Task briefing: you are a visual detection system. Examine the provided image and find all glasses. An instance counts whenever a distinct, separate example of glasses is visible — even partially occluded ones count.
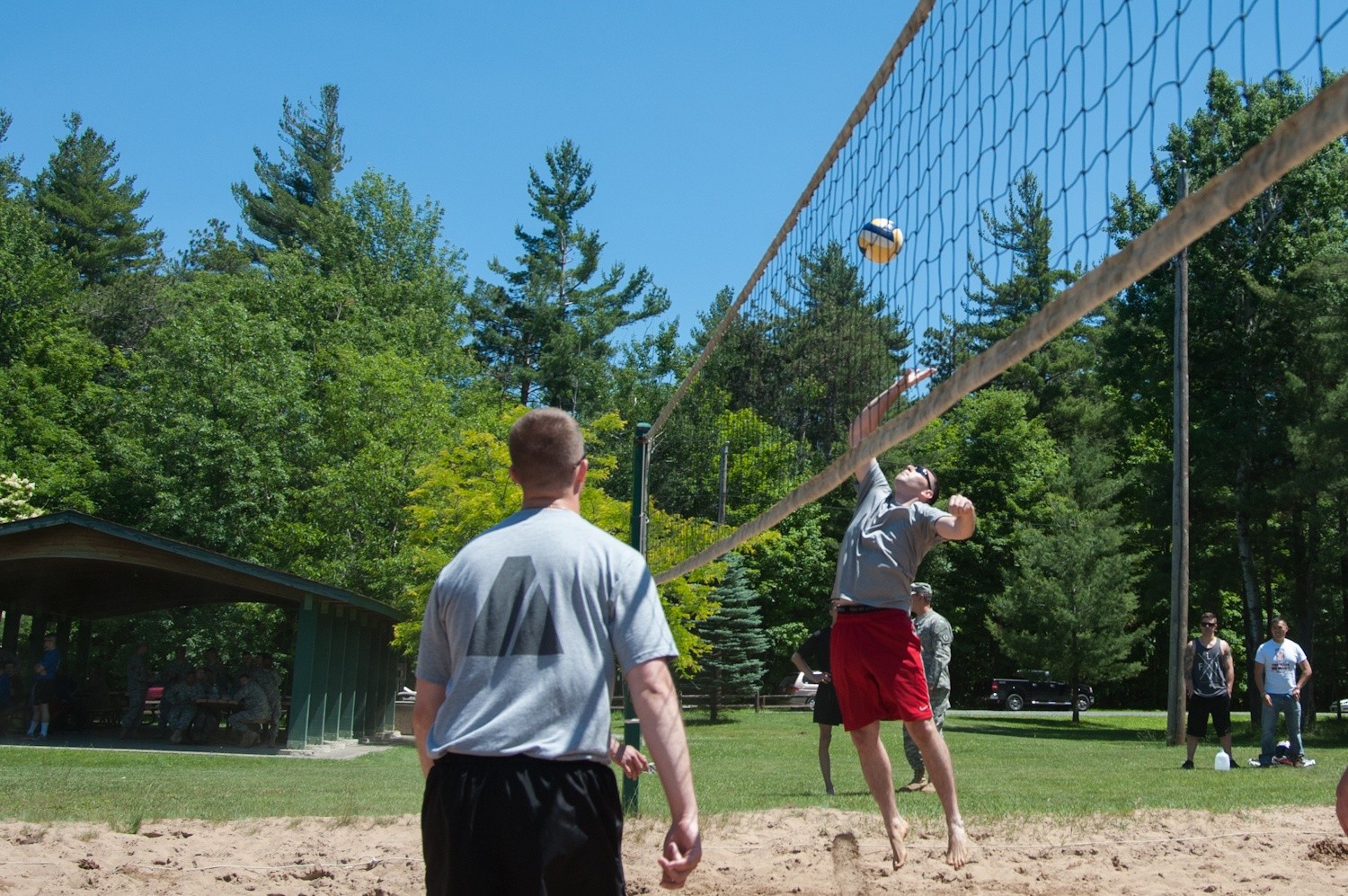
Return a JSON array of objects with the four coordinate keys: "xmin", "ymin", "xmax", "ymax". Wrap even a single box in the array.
[
  {"xmin": 913, "ymin": 464, "xmax": 931, "ymax": 490},
  {"xmin": 1201, "ymin": 622, "xmax": 1216, "ymax": 627}
]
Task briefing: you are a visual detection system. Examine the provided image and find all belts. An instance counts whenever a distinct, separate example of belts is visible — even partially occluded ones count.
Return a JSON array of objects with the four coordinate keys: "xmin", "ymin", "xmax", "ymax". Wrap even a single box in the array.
[{"xmin": 837, "ymin": 604, "xmax": 883, "ymax": 614}]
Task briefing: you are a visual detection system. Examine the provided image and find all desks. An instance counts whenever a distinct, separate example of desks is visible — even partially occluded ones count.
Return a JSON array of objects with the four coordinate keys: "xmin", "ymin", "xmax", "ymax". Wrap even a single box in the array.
[{"xmin": 195, "ymin": 699, "xmax": 237, "ymax": 742}]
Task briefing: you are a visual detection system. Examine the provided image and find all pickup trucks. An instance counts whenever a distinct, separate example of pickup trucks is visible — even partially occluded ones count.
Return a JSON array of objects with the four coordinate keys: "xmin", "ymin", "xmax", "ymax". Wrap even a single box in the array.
[{"xmin": 973, "ymin": 668, "xmax": 1096, "ymax": 711}]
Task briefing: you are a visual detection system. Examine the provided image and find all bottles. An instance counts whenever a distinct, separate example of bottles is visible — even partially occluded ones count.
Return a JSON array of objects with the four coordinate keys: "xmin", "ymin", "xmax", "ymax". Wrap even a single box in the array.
[
  {"xmin": 1214, "ymin": 748, "xmax": 1231, "ymax": 771},
  {"xmin": 209, "ymin": 681, "xmax": 233, "ymax": 699}
]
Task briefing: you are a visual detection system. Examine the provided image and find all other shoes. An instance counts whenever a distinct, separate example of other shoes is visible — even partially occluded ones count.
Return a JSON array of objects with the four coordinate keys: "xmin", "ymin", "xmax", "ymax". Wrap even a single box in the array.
[
  {"xmin": 1230, "ymin": 760, "xmax": 1240, "ymax": 768},
  {"xmin": 1182, "ymin": 760, "xmax": 1194, "ymax": 770},
  {"xmin": 1294, "ymin": 756, "xmax": 1306, "ymax": 767},
  {"xmin": 1259, "ymin": 764, "xmax": 1270, "ymax": 768}
]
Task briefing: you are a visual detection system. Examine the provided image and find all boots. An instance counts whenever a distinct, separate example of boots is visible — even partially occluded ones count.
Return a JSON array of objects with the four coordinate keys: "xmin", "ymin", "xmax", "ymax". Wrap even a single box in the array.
[
  {"xmin": 899, "ymin": 769, "xmax": 928, "ymax": 792},
  {"xmin": 921, "ymin": 781, "xmax": 937, "ymax": 792}
]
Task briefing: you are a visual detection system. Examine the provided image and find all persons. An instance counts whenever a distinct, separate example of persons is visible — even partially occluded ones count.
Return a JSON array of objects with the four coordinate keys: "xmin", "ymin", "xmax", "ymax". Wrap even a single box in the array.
[
  {"xmin": 0, "ymin": 631, "xmax": 282, "ymax": 750},
  {"xmin": 827, "ymin": 364, "xmax": 977, "ymax": 871},
  {"xmin": 1335, "ymin": 766, "xmax": 1348, "ymax": 836},
  {"xmin": 792, "ymin": 581, "xmax": 955, "ymax": 795},
  {"xmin": 1181, "ymin": 611, "xmax": 1243, "ymax": 770},
  {"xmin": 1257, "ymin": 614, "xmax": 1316, "ymax": 767},
  {"xmin": 414, "ymin": 407, "xmax": 702, "ymax": 896}
]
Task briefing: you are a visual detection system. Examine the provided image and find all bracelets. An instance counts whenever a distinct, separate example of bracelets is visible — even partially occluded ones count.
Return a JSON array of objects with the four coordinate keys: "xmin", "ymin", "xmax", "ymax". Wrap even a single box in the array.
[{"xmin": 1261, "ymin": 691, "xmax": 1267, "ymax": 698}]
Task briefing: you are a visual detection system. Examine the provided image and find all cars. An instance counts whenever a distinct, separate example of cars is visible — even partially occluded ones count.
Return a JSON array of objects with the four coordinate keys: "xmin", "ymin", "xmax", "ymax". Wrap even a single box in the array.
[
  {"xmin": 775, "ymin": 670, "xmax": 823, "ymax": 711},
  {"xmin": 396, "ymin": 686, "xmax": 417, "ymax": 702},
  {"xmin": 1329, "ymin": 697, "xmax": 1348, "ymax": 713}
]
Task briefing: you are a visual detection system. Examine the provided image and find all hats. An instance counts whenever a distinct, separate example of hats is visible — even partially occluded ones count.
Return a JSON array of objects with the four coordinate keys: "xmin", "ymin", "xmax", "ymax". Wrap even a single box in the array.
[{"xmin": 910, "ymin": 583, "xmax": 932, "ymax": 599}]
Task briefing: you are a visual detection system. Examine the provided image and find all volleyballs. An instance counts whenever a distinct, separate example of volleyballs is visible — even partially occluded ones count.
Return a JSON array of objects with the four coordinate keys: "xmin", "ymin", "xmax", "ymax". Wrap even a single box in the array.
[{"xmin": 860, "ymin": 220, "xmax": 901, "ymax": 261}]
